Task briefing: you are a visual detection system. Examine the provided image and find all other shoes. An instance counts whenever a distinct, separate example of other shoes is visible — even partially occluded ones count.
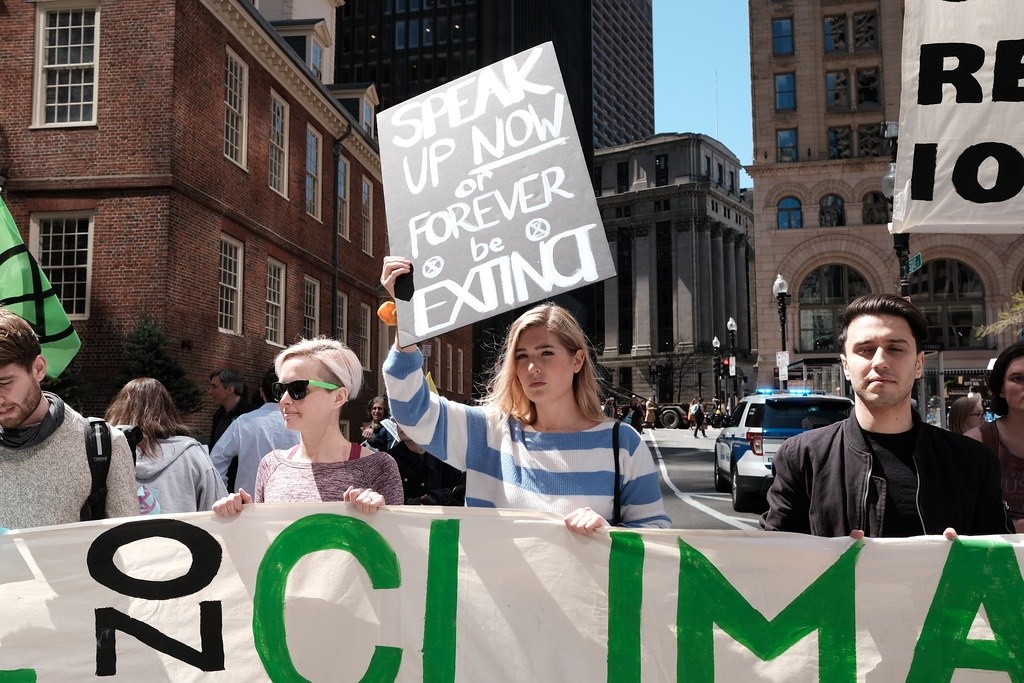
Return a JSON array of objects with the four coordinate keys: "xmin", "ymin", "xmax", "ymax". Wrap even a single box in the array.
[
  {"xmin": 651, "ymin": 426, "xmax": 655, "ymax": 430},
  {"xmin": 693, "ymin": 435, "xmax": 699, "ymax": 439},
  {"xmin": 702, "ymin": 435, "xmax": 708, "ymax": 439}
]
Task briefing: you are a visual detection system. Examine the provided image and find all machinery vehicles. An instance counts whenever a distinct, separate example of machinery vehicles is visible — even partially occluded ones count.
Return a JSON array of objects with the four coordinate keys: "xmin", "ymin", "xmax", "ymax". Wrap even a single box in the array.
[{"xmin": 638, "ymin": 399, "xmax": 689, "ymax": 428}]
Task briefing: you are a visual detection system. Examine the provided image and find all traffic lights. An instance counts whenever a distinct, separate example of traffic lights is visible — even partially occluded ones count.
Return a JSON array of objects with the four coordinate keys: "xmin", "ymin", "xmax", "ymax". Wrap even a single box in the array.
[{"xmin": 722, "ymin": 360, "xmax": 729, "ymax": 377}]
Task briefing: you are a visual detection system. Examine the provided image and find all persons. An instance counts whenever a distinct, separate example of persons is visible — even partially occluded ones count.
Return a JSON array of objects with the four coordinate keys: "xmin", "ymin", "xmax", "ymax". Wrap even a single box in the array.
[
  {"xmin": 0, "ymin": 307, "xmax": 140, "ymax": 530},
  {"xmin": 380, "ymin": 256, "xmax": 673, "ymax": 536},
  {"xmin": 209, "ymin": 368, "xmax": 300, "ymax": 503},
  {"xmin": 212, "ymin": 339, "xmax": 404, "ymax": 516},
  {"xmin": 949, "ymin": 341, "xmax": 1024, "ymax": 533},
  {"xmin": 104, "ymin": 377, "xmax": 229, "ymax": 515},
  {"xmin": 759, "ymin": 292, "xmax": 1016, "ymax": 539},
  {"xmin": 688, "ymin": 397, "xmax": 708, "ymax": 438},
  {"xmin": 360, "ymin": 398, "xmax": 467, "ymax": 506},
  {"xmin": 604, "ymin": 395, "xmax": 656, "ymax": 435}
]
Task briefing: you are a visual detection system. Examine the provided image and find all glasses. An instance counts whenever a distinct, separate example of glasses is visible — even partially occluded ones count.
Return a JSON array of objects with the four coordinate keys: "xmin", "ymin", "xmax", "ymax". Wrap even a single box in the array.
[
  {"xmin": 272, "ymin": 379, "xmax": 348, "ymax": 403},
  {"xmin": 969, "ymin": 412, "xmax": 987, "ymax": 419}
]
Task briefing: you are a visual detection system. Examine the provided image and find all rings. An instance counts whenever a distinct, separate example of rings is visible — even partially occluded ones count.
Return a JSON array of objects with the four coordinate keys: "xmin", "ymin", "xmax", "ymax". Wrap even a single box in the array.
[{"xmin": 367, "ymin": 496, "xmax": 374, "ymax": 502}]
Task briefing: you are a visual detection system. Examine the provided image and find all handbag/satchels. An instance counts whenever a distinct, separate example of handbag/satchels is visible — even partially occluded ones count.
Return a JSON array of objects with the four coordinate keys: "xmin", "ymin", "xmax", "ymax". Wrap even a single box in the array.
[
  {"xmin": 691, "ymin": 404, "xmax": 700, "ymax": 416},
  {"xmin": 688, "ymin": 412, "xmax": 696, "ymax": 421}
]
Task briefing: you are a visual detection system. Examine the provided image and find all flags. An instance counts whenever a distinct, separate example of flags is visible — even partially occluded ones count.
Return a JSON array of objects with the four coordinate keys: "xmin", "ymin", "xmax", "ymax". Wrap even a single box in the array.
[{"xmin": 0, "ymin": 196, "xmax": 82, "ymax": 380}]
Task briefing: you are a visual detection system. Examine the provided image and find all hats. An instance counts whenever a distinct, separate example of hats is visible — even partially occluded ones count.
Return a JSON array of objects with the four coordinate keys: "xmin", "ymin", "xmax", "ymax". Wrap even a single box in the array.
[{"xmin": 609, "ymin": 397, "xmax": 614, "ymax": 401}]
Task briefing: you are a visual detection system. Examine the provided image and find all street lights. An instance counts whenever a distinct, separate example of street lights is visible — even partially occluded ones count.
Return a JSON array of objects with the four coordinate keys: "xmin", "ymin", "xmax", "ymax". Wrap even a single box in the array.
[
  {"xmin": 712, "ymin": 336, "xmax": 721, "ymax": 398},
  {"xmin": 882, "ymin": 163, "xmax": 920, "ymax": 412},
  {"xmin": 772, "ymin": 272, "xmax": 789, "ymax": 390},
  {"xmin": 727, "ymin": 317, "xmax": 738, "ymax": 413}
]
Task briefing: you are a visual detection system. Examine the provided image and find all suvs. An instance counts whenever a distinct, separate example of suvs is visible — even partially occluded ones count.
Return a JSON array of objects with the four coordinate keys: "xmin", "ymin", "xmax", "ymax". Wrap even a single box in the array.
[{"xmin": 714, "ymin": 388, "xmax": 856, "ymax": 511}]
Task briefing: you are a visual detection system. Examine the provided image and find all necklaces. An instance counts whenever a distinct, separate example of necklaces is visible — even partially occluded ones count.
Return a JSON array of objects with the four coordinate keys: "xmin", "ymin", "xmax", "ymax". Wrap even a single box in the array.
[{"xmin": 536, "ymin": 413, "xmax": 585, "ymax": 429}]
